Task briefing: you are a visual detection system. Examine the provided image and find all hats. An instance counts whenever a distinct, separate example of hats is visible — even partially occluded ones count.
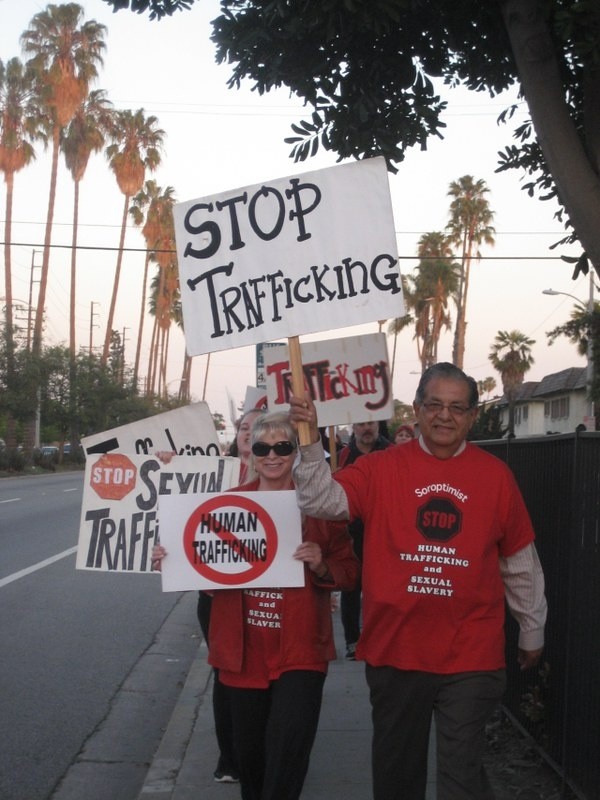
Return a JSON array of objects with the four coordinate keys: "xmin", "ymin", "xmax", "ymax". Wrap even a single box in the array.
[{"xmin": 395, "ymin": 424, "xmax": 415, "ymax": 434}]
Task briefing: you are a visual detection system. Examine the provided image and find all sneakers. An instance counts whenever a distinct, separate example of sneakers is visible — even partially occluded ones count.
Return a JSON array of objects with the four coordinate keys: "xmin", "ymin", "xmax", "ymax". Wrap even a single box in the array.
[
  {"xmin": 213, "ymin": 754, "xmax": 239, "ymax": 782},
  {"xmin": 345, "ymin": 641, "xmax": 359, "ymax": 660}
]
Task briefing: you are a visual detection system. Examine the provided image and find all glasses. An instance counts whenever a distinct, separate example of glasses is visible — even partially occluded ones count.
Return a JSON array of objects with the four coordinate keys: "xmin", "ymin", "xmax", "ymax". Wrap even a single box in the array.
[{"xmin": 252, "ymin": 441, "xmax": 295, "ymax": 456}]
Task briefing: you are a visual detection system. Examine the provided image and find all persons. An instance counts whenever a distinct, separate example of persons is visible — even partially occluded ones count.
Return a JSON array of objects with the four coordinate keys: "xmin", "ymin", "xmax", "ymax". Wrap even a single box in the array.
[
  {"xmin": 318, "ymin": 426, "xmax": 350, "ymax": 459},
  {"xmin": 393, "ymin": 424, "xmax": 416, "ymax": 445},
  {"xmin": 151, "ymin": 413, "xmax": 360, "ymax": 800},
  {"xmin": 287, "ymin": 362, "xmax": 550, "ymax": 800},
  {"xmin": 156, "ymin": 409, "xmax": 268, "ymax": 784},
  {"xmin": 324, "ymin": 420, "xmax": 395, "ymax": 660}
]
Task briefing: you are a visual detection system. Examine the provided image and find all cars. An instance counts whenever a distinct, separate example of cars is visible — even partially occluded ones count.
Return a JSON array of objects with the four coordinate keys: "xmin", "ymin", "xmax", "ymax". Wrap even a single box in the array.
[{"xmin": 0, "ymin": 439, "xmax": 82, "ymax": 462}]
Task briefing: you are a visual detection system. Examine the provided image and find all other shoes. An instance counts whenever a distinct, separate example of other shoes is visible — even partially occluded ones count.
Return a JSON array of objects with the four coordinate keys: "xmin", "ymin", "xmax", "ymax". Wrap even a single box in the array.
[{"xmin": 331, "ymin": 593, "xmax": 338, "ymax": 610}]
